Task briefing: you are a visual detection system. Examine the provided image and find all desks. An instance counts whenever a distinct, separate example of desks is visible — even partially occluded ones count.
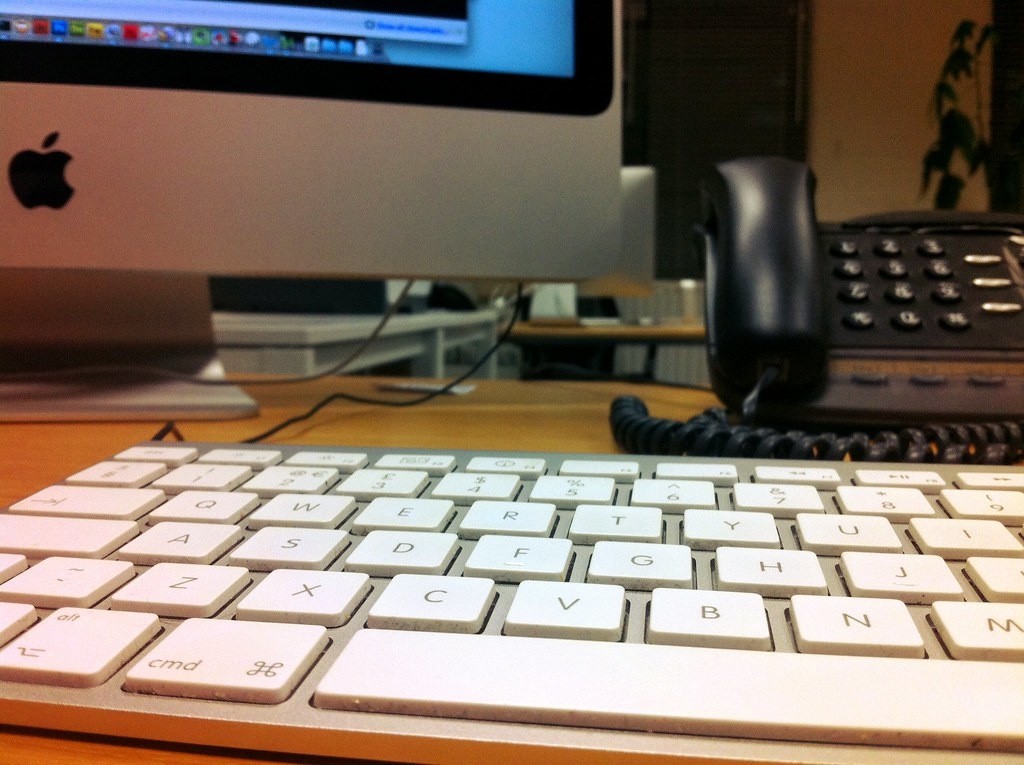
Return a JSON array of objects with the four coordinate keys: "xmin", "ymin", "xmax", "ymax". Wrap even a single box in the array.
[
  {"xmin": 213, "ymin": 312, "xmax": 498, "ymax": 380},
  {"xmin": 498, "ymin": 316, "xmax": 706, "ymax": 380},
  {"xmin": 1, "ymin": 375, "xmax": 1024, "ymax": 765}
]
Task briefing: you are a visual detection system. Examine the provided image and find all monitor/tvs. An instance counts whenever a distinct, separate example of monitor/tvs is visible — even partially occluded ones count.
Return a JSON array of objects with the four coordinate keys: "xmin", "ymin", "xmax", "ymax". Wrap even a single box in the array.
[
  {"xmin": 0, "ymin": 0, "xmax": 617, "ymax": 426},
  {"xmin": 448, "ymin": 166, "xmax": 656, "ymax": 325}
]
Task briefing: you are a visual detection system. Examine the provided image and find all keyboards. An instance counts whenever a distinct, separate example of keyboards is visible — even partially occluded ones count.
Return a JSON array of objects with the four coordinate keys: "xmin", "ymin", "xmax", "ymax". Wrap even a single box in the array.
[{"xmin": 0, "ymin": 444, "xmax": 1024, "ymax": 765}]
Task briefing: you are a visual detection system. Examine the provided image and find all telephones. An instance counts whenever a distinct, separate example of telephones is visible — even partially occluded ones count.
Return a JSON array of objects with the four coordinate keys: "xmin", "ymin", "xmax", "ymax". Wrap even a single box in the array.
[{"xmin": 699, "ymin": 152, "xmax": 1024, "ymax": 431}]
